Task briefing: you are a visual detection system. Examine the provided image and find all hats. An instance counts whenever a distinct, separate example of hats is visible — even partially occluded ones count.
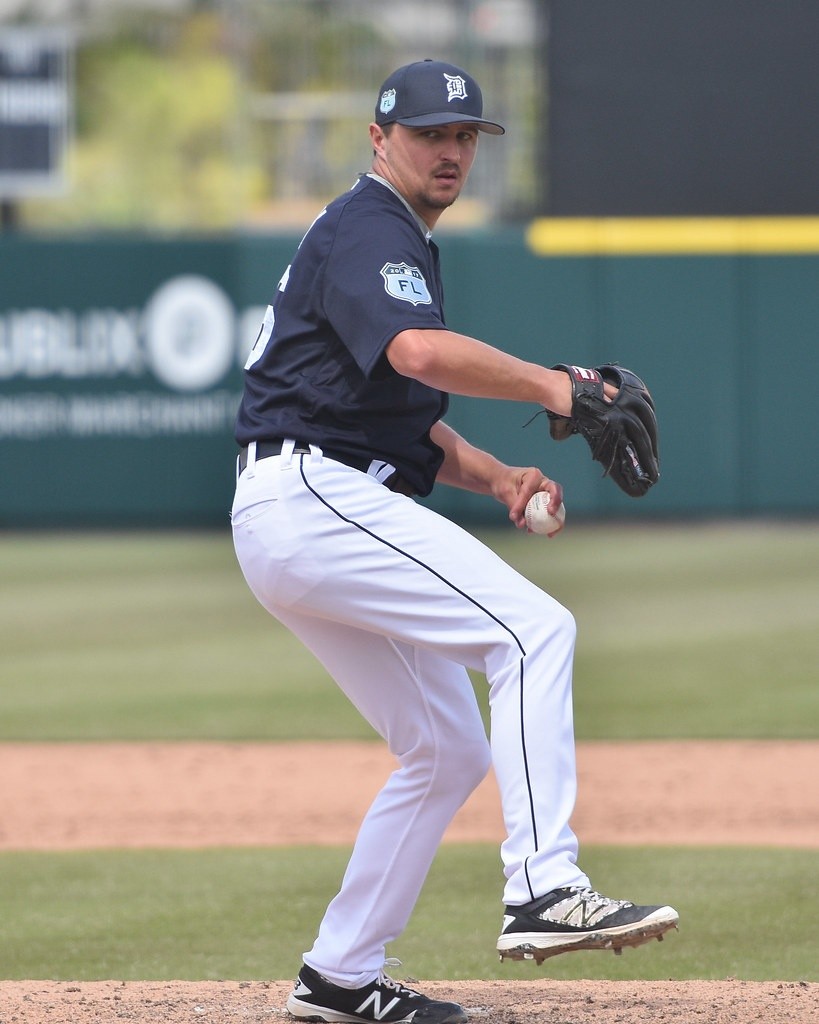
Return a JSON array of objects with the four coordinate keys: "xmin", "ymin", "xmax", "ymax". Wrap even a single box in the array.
[{"xmin": 376, "ymin": 58, "xmax": 505, "ymax": 136}]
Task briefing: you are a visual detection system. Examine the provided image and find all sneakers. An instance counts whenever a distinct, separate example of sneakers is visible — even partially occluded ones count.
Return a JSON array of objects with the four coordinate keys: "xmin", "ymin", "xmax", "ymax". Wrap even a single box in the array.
[
  {"xmin": 496, "ymin": 883, "xmax": 679, "ymax": 965},
  {"xmin": 286, "ymin": 948, "xmax": 467, "ymax": 1024}
]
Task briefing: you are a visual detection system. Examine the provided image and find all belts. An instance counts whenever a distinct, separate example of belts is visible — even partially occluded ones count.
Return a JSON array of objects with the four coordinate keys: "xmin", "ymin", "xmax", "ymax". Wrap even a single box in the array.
[{"xmin": 238, "ymin": 437, "xmax": 416, "ymax": 499}]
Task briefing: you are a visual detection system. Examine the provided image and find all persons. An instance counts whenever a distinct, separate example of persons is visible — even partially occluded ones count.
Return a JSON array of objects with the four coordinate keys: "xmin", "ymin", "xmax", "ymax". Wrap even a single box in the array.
[{"xmin": 231, "ymin": 59, "xmax": 680, "ymax": 1024}]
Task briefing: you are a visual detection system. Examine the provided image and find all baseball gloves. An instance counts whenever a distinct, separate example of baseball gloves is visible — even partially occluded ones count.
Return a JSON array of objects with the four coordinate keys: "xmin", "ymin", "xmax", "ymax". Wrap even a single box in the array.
[{"xmin": 521, "ymin": 360, "xmax": 663, "ymax": 499}]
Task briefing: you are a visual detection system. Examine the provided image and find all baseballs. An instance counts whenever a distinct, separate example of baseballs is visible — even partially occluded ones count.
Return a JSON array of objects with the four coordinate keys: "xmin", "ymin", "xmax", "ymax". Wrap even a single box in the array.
[{"xmin": 523, "ymin": 491, "xmax": 567, "ymax": 536}]
